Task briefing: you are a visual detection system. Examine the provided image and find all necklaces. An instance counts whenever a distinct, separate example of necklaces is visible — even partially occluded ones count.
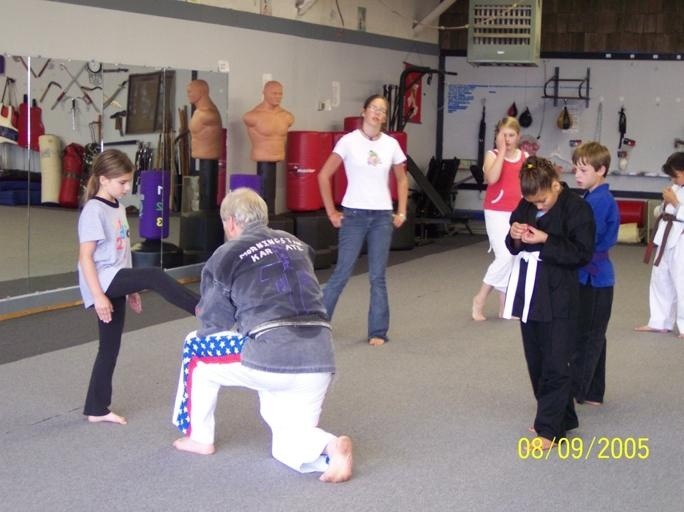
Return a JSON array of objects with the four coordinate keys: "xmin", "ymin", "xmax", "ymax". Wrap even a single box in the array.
[{"xmin": 360, "ymin": 127, "xmax": 383, "ymax": 141}]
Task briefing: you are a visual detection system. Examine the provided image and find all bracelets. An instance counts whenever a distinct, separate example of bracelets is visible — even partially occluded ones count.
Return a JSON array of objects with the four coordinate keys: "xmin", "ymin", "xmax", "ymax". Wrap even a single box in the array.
[{"xmin": 397, "ymin": 211, "xmax": 407, "ymax": 220}]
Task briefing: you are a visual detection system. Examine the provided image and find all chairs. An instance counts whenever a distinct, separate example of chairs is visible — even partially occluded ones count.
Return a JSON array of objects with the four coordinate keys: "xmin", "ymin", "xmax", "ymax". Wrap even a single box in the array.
[{"xmin": 408, "ymin": 156, "xmax": 461, "ymax": 239}]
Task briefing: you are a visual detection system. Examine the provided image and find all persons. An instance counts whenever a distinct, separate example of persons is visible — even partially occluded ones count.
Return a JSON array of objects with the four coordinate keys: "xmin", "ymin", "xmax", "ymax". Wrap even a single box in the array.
[
  {"xmin": 186, "ymin": 79, "xmax": 223, "ymax": 161},
  {"xmin": 572, "ymin": 141, "xmax": 621, "ymax": 405},
  {"xmin": 76, "ymin": 147, "xmax": 203, "ymax": 426},
  {"xmin": 504, "ymin": 155, "xmax": 595, "ymax": 454},
  {"xmin": 170, "ymin": 186, "xmax": 353, "ymax": 482},
  {"xmin": 633, "ymin": 150, "xmax": 684, "ymax": 339},
  {"xmin": 242, "ymin": 80, "xmax": 295, "ymax": 163},
  {"xmin": 471, "ymin": 116, "xmax": 531, "ymax": 322},
  {"xmin": 316, "ymin": 94, "xmax": 410, "ymax": 347}
]
